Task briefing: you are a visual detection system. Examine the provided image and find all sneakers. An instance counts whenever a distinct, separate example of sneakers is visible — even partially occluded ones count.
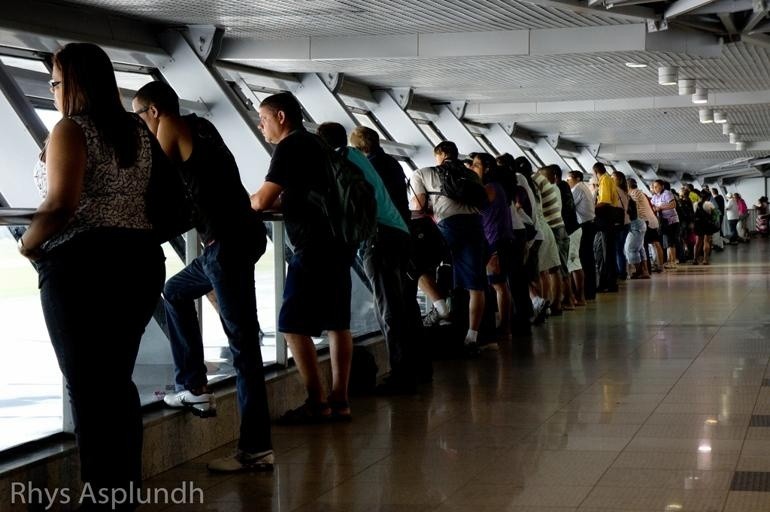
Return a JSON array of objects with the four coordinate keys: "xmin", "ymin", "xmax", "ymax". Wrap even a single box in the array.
[
  {"xmin": 164, "ymin": 390, "xmax": 216, "ymax": 417},
  {"xmin": 369, "ymin": 374, "xmax": 434, "ymax": 395},
  {"xmin": 207, "ymin": 449, "xmax": 275, "ymax": 473},
  {"xmin": 530, "ymin": 297, "xmax": 550, "ymax": 324},
  {"xmin": 422, "ymin": 308, "xmax": 453, "ymax": 327},
  {"xmin": 452, "ymin": 329, "xmax": 512, "ymax": 355}
]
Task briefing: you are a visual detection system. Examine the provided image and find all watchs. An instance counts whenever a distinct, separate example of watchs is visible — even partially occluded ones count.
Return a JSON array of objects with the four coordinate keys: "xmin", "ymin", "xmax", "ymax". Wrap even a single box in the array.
[{"xmin": 17, "ymin": 238, "xmax": 30, "ymax": 255}]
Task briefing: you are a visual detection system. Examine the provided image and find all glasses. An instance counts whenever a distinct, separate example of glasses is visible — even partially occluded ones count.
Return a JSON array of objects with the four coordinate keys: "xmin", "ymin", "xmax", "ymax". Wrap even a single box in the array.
[{"xmin": 50, "ymin": 79, "xmax": 63, "ymax": 87}]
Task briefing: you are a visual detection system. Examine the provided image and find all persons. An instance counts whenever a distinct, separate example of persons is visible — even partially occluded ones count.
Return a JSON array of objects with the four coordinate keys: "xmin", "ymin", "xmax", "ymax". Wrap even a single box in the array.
[
  {"xmin": 248, "ymin": 90, "xmax": 358, "ymax": 425},
  {"xmin": 316, "ymin": 120, "xmax": 770, "ymax": 400},
  {"xmin": 14, "ymin": 42, "xmax": 168, "ymax": 511},
  {"xmin": 131, "ymin": 79, "xmax": 276, "ymax": 474}
]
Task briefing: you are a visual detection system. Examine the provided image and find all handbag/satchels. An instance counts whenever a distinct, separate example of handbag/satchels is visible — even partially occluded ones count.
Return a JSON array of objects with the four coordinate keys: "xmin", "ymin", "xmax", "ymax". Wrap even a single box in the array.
[
  {"xmin": 595, "ymin": 206, "xmax": 625, "ymax": 226},
  {"xmin": 657, "ymin": 216, "xmax": 668, "ymax": 234},
  {"xmin": 705, "ymin": 221, "xmax": 720, "ymax": 233},
  {"xmin": 143, "ymin": 160, "xmax": 201, "ymax": 241},
  {"xmin": 486, "ymin": 256, "xmax": 498, "ymax": 274},
  {"xmin": 392, "ymin": 215, "xmax": 449, "ymax": 280}
]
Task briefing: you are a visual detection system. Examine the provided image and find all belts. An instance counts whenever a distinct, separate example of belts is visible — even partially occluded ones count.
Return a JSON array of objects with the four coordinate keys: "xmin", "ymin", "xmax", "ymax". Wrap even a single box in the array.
[{"xmin": 580, "ymin": 219, "xmax": 595, "ymax": 226}]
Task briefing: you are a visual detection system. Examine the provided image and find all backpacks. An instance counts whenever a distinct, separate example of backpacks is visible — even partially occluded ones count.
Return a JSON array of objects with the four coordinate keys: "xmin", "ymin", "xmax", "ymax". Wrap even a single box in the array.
[
  {"xmin": 627, "ymin": 196, "xmax": 637, "ymax": 220},
  {"xmin": 294, "ymin": 131, "xmax": 377, "ymax": 247},
  {"xmin": 436, "ymin": 157, "xmax": 489, "ymax": 208}
]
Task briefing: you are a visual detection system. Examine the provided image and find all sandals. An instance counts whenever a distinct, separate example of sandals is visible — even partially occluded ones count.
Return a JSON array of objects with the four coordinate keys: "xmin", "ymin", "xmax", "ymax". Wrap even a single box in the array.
[{"xmin": 284, "ymin": 400, "xmax": 353, "ymax": 424}]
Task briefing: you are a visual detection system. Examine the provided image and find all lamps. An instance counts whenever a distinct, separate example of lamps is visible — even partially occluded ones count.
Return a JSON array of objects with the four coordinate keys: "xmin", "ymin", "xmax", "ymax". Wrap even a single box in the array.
[
  {"xmin": 735, "ymin": 142, "xmax": 746, "ymax": 152},
  {"xmin": 712, "ymin": 112, "xmax": 727, "ymax": 124},
  {"xmin": 692, "ymin": 88, "xmax": 708, "ymax": 104},
  {"xmin": 722, "ymin": 125, "xmax": 734, "ymax": 135},
  {"xmin": 657, "ymin": 66, "xmax": 678, "ymax": 86},
  {"xmin": 699, "ymin": 109, "xmax": 713, "ymax": 123},
  {"xmin": 676, "ymin": 79, "xmax": 696, "ymax": 96},
  {"xmin": 729, "ymin": 134, "xmax": 739, "ymax": 144}
]
